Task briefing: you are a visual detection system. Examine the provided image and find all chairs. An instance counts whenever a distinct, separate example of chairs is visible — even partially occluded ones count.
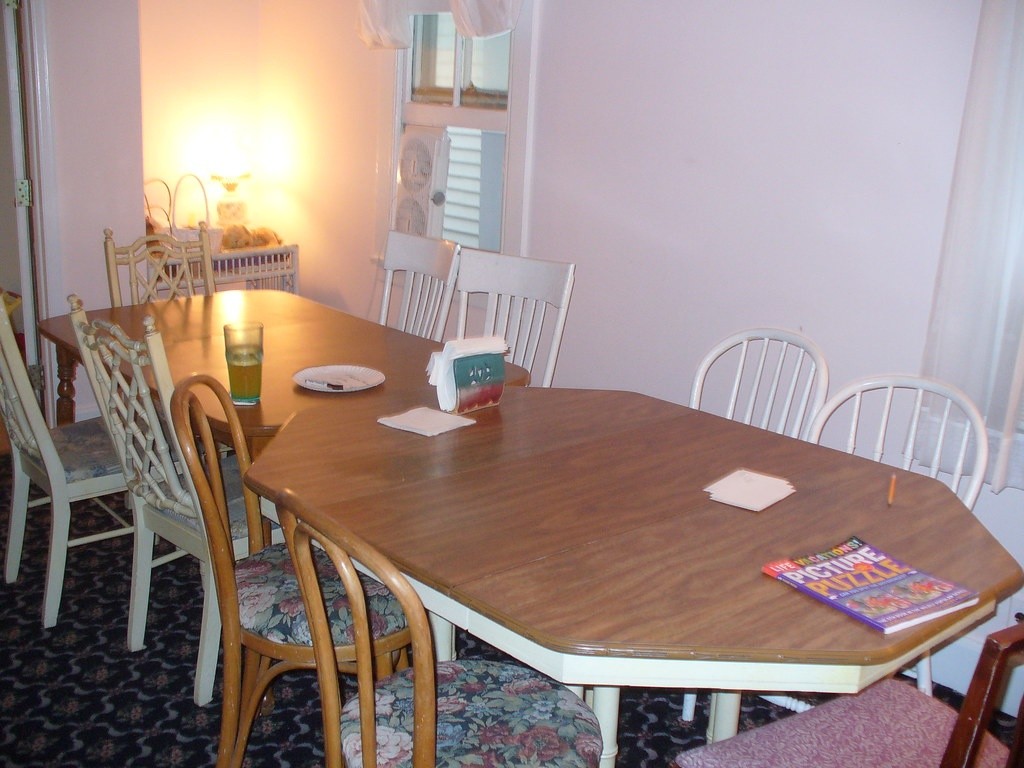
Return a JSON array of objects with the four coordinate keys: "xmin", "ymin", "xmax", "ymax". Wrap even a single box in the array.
[
  {"xmin": 272, "ymin": 487, "xmax": 604, "ymax": 768},
  {"xmin": 681, "ymin": 325, "xmax": 990, "ymax": 722},
  {"xmin": 169, "ymin": 373, "xmax": 413, "ymax": 768},
  {"xmin": 66, "ymin": 294, "xmax": 283, "ymax": 706},
  {"xmin": 0, "ymin": 293, "xmax": 230, "ymax": 630},
  {"xmin": 104, "ymin": 221, "xmax": 216, "ymax": 309},
  {"xmin": 667, "ymin": 617, "xmax": 1024, "ymax": 768},
  {"xmin": 376, "ymin": 228, "xmax": 576, "ymax": 388}
]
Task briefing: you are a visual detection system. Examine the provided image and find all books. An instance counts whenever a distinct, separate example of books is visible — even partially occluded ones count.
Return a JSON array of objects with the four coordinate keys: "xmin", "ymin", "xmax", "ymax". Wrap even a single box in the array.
[{"xmin": 761, "ymin": 535, "xmax": 979, "ymax": 634}]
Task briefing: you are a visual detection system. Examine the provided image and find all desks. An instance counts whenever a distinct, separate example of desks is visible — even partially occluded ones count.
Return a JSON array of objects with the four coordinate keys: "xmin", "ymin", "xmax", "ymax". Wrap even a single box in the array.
[
  {"xmin": 34, "ymin": 291, "xmax": 532, "ymax": 548},
  {"xmin": 240, "ymin": 388, "xmax": 1023, "ymax": 768}
]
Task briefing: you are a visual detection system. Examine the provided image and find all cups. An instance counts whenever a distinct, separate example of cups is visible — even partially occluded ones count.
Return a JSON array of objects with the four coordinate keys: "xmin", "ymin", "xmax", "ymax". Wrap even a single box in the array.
[{"xmin": 223, "ymin": 321, "xmax": 263, "ymax": 405}]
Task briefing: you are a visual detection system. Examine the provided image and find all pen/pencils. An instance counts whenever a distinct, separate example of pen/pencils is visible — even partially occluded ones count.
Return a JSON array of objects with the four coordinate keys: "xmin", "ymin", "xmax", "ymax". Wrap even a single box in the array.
[{"xmin": 887, "ymin": 474, "xmax": 897, "ymax": 508}]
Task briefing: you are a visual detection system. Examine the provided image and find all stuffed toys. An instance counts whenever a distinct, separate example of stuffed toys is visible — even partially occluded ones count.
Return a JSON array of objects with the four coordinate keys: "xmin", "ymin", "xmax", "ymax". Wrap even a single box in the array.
[{"xmin": 219, "ymin": 223, "xmax": 282, "ymax": 251}]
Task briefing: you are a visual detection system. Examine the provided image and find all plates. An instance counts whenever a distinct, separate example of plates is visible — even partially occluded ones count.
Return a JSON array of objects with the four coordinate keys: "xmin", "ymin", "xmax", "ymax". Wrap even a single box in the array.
[{"xmin": 292, "ymin": 364, "xmax": 386, "ymax": 393}]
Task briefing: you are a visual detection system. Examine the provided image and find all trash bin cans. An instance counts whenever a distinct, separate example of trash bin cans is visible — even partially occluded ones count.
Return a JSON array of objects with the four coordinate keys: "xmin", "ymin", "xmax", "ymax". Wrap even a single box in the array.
[{"xmin": 0, "ymin": 288, "xmax": 27, "ymax": 456}]
